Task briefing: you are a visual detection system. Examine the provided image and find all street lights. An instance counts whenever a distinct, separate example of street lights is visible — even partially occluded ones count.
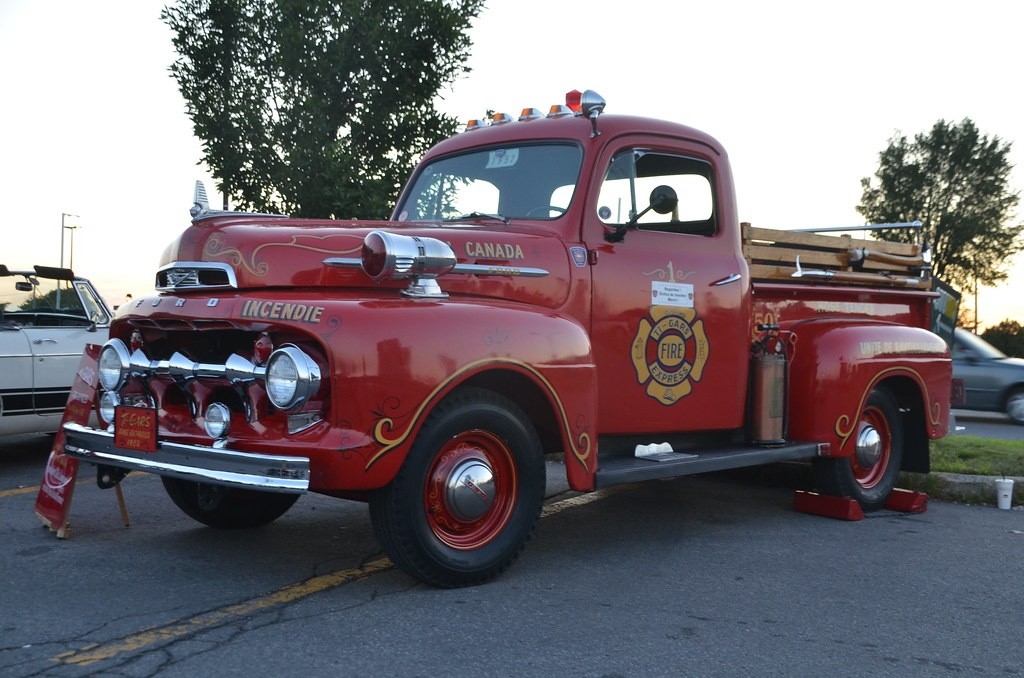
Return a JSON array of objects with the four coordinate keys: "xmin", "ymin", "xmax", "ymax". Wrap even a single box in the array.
[{"xmin": 63, "ymin": 212, "xmax": 81, "ymax": 274}]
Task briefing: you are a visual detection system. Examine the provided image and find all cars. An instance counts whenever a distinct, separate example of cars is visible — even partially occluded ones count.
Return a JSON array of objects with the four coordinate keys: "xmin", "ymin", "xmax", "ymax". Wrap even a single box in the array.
[
  {"xmin": 947, "ymin": 322, "xmax": 1024, "ymax": 423},
  {"xmin": 1, "ymin": 265, "xmax": 114, "ymax": 445}
]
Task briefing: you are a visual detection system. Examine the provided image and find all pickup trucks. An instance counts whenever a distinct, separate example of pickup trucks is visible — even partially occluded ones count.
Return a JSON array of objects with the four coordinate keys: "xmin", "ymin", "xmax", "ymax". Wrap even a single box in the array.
[{"xmin": 63, "ymin": 86, "xmax": 957, "ymax": 590}]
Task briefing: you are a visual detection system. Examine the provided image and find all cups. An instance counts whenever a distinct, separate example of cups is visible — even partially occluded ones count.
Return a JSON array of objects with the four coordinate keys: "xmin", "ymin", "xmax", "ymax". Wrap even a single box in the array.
[{"xmin": 995, "ymin": 479, "xmax": 1014, "ymax": 509}]
